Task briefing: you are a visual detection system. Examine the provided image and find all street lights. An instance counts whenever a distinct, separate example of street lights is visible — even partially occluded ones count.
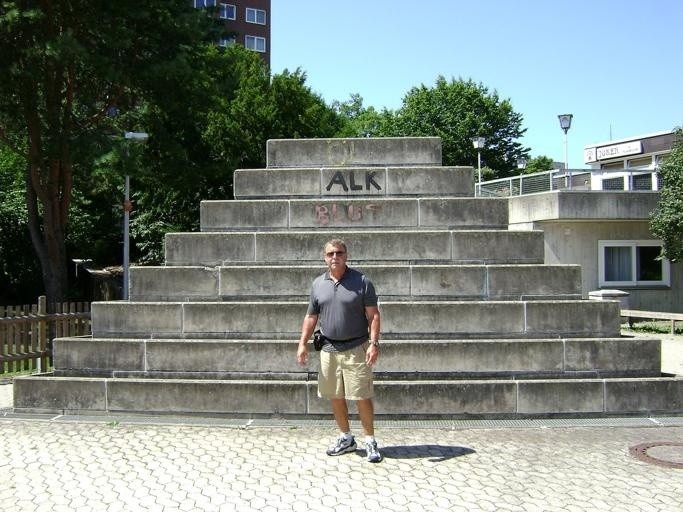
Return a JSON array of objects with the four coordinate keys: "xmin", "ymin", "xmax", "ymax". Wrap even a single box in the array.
[
  {"xmin": 472, "ymin": 135, "xmax": 485, "ymax": 195},
  {"xmin": 558, "ymin": 114, "xmax": 572, "ymax": 187},
  {"xmin": 516, "ymin": 156, "xmax": 526, "ymax": 196}
]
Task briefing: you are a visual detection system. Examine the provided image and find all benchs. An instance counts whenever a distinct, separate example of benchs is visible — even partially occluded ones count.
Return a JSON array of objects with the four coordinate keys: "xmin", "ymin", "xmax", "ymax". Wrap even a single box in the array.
[{"xmin": 618, "ymin": 310, "xmax": 683, "ymax": 335}]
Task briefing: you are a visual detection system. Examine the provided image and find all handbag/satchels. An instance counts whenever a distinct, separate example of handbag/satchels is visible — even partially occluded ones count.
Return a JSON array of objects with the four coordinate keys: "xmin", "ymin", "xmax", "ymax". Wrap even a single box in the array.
[{"xmin": 314, "ymin": 329, "xmax": 324, "ymax": 351}]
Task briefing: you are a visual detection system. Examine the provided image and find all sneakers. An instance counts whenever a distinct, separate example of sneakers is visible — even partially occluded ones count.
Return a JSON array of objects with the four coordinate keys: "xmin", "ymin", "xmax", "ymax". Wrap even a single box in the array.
[
  {"xmin": 365, "ymin": 441, "xmax": 382, "ymax": 462},
  {"xmin": 326, "ymin": 435, "xmax": 357, "ymax": 456}
]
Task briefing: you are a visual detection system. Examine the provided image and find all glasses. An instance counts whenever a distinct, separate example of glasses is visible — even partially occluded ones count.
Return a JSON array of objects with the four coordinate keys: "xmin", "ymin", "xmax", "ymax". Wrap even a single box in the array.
[{"xmin": 325, "ymin": 251, "xmax": 346, "ymax": 256}]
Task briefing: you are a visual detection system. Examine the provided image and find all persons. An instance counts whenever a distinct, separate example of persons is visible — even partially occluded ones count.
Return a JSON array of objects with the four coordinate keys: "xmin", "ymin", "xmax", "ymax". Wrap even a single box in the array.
[{"xmin": 295, "ymin": 239, "xmax": 382, "ymax": 462}]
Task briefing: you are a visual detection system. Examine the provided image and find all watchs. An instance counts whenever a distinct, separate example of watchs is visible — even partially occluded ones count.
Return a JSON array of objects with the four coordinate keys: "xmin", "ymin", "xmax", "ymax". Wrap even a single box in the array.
[{"xmin": 367, "ymin": 339, "xmax": 380, "ymax": 348}]
